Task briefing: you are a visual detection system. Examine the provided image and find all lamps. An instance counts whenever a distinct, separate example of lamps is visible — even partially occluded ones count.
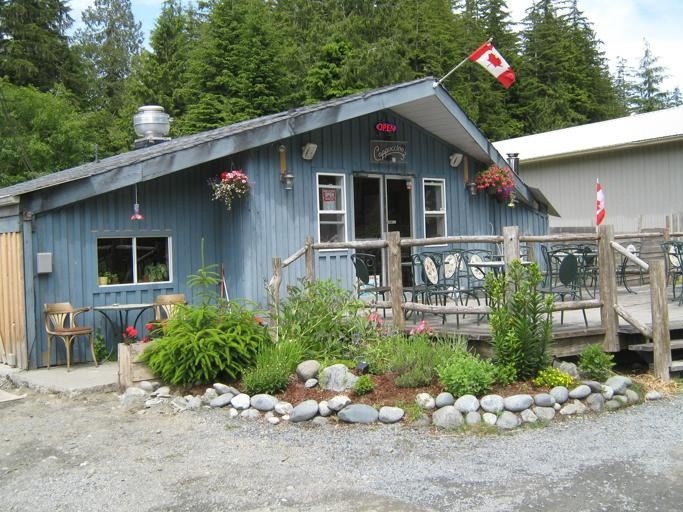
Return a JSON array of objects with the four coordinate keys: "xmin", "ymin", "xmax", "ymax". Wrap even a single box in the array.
[
  {"xmin": 463, "ymin": 176, "xmax": 477, "ymax": 197},
  {"xmin": 280, "ymin": 169, "xmax": 297, "ymax": 193},
  {"xmin": 507, "ymin": 192, "xmax": 518, "ymax": 208},
  {"xmin": 130, "ymin": 181, "xmax": 144, "ymax": 222}
]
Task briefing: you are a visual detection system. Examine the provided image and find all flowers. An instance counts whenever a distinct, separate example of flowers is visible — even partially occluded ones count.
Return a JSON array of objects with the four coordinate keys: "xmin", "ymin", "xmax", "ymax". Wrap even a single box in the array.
[
  {"xmin": 468, "ymin": 161, "xmax": 515, "ymax": 197},
  {"xmin": 115, "ymin": 322, "xmax": 155, "ymax": 346},
  {"xmin": 206, "ymin": 168, "xmax": 251, "ymax": 210}
]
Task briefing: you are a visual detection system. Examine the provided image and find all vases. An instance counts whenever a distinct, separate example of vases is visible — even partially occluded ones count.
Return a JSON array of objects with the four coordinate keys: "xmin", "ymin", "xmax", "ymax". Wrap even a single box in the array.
[
  {"xmin": 98, "ymin": 277, "xmax": 107, "ymax": 285},
  {"xmin": 487, "ymin": 187, "xmax": 497, "ymax": 197}
]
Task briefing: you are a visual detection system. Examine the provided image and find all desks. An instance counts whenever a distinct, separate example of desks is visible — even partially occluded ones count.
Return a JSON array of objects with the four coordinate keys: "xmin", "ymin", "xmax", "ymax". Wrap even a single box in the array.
[{"xmin": 92, "ymin": 303, "xmax": 159, "ymax": 368}]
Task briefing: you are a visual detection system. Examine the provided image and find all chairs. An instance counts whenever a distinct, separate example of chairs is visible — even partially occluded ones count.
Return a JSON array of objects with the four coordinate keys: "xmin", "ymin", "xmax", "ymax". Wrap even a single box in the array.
[
  {"xmin": 540, "ymin": 243, "xmax": 599, "ymax": 328},
  {"xmin": 660, "ymin": 241, "xmax": 683, "ymax": 301},
  {"xmin": 404, "ymin": 249, "xmax": 494, "ymax": 325},
  {"xmin": 350, "ymin": 253, "xmax": 392, "ymax": 318},
  {"xmin": 38, "ymin": 297, "xmax": 98, "ymax": 370},
  {"xmin": 153, "ymin": 291, "xmax": 186, "ymax": 325},
  {"xmin": 614, "ymin": 241, "xmax": 645, "ymax": 286}
]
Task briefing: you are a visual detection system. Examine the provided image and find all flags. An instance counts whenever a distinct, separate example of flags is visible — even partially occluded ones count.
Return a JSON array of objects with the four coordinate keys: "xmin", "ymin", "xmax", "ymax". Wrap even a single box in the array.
[
  {"xmin": 469, "ymin": 40, "xmax": 515, "ymax": 90},
  {"xmin": 596, "ymin": 181, "xmax": 606, "ymax": 227}
]
